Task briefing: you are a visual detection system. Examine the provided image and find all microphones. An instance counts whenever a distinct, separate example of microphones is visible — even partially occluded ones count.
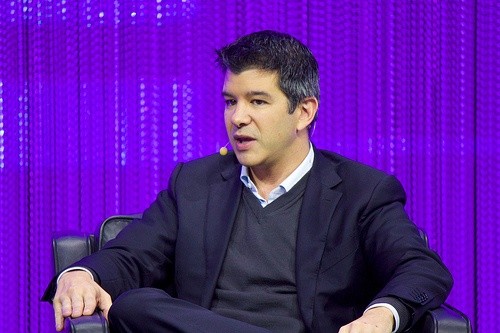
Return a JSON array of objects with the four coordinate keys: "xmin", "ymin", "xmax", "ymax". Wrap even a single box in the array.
[{"xmin": 220, "ymin": 141, "xmax": 232, "ymax": 157}]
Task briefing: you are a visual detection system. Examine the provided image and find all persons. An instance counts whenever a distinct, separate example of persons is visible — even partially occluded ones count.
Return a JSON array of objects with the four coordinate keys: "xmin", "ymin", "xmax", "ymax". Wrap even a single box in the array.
[{"xmin": 41, "ymin": 29, "xmax": 453, "ymax": 333}]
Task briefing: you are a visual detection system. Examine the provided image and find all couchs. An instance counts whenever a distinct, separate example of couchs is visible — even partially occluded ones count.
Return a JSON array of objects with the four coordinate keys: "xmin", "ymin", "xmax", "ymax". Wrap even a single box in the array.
[{"xmin": 52, "ymin": 213, "xmax": 475, "ymax": 333}]
